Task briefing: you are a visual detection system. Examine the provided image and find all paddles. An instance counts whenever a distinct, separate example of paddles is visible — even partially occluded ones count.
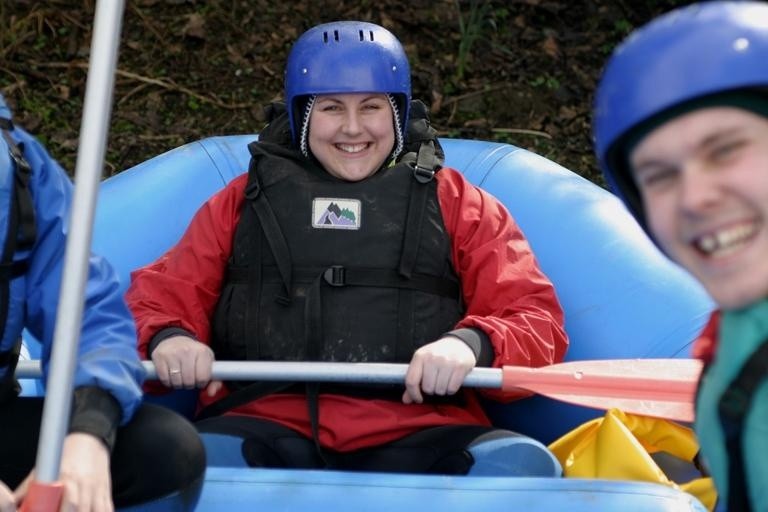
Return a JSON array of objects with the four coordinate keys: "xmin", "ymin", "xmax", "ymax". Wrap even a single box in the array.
[{"xmin": 12, "ymin": 358, "xmax": 703, "ymax": 425}]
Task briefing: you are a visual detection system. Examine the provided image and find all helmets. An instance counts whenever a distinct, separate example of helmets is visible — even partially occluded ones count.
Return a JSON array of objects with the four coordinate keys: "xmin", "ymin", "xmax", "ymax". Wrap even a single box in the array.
[
  {"xmin": 285, "ymin": 21, "xmax": 411, "ymax": 161},
  {"xmin": 592, "ymin": 0, "xmax": 768, "ymax": 262}
]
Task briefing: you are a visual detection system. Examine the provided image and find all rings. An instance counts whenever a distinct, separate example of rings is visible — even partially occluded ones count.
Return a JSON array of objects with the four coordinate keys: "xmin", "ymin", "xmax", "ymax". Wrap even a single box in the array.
[{"xmin": 170, "ymin": 369, "xmax": 181, "ymax": 374}]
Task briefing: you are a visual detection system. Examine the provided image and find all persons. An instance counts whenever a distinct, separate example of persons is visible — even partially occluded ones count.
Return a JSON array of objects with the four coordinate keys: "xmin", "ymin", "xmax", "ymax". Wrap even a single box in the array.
[
  {"xmin": 0, "ymin": 95, "xmax": 207, "ymax": 512},
  {"xmin": 122, "ymin": 20, "xmax": 571, "ymax": 476},
  {"xmin": 587, "ymin": 0, "xmax": 768, "ymax": 512}
]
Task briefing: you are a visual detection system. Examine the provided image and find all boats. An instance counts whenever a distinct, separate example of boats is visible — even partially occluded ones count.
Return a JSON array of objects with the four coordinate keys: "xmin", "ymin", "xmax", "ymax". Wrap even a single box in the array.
[{"xmin": 0, "ymin": 134, "xmax": 727, "ymax": 512}]
